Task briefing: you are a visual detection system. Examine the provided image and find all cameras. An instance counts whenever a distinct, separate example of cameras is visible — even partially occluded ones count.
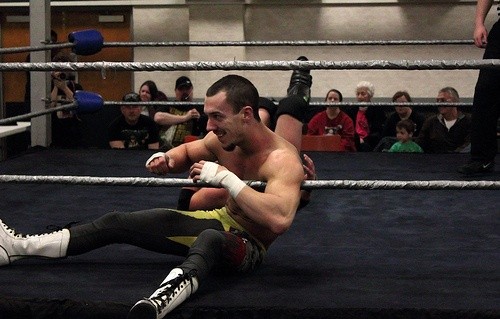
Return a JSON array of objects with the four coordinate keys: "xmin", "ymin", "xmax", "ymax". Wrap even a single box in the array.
[{"xmin": 58, "ymin": 72, "xmax": 67, "ymax": 80}]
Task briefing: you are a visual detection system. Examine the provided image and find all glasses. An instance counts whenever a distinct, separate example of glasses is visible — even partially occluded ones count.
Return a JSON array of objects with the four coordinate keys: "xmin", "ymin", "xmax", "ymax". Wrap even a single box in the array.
[{"xmin": 436, "ymin": 97, "xmax": 452, "ymax": 103}]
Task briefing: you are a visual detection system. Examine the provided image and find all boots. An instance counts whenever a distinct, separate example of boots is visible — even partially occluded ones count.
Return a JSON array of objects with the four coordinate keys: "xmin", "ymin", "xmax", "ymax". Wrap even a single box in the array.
[
  {"xmin": 0, "ymin": 220, "xmax": 70, "ymax": 263},
  {"xmin": 129, "ymin": 268, "xmax": 199, "ymax": 319}
]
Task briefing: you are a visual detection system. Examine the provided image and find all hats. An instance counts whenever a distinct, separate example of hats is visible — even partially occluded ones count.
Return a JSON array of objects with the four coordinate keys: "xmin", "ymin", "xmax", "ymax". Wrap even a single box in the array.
[
  {"xmin": 176, "ymin": 76, "xmax": 192, "ymax": 89},
  {"xmin": 123, "ymin": 92, "xmax": 142, "ymax": 102}
]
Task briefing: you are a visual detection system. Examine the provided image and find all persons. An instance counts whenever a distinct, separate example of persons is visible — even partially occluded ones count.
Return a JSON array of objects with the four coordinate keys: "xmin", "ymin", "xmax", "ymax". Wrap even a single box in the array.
[
  {"xmin": 24, "ymin": 0, "xmax": 500, "ymax": 212},
  {"xmin": 0, "ymin": 75, "xmax": 306, "ymax": 319}
]
